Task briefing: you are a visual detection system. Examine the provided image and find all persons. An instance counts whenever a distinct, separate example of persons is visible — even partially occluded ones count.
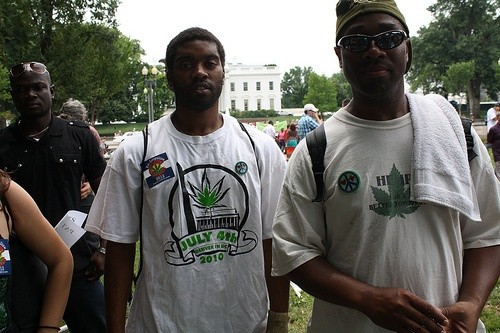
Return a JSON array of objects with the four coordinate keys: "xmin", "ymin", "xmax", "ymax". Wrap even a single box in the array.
[
  {"xmin": 84, "ymin": 27, "xmax": 290, "ymax": 333},
  {"xmin": 487, "ymin": 103, "xmax": 500, "ymax": 133},
  {"xmin": 270, "ymin": 0, "xmax": 500, "ymax": 333},
  {"xmin": 263, "ymin": 120, "xmax": 276, "ymax": 137},
  {"xmin": 279, "ymin": 123, "xmax": 298, "ymax": 152},
  {"xmin": 0, "ymin": 169, "xmax": 74, "ymax": 333},
  {"xmin": 60, "ymin": 100, "xmax": 104, "ymax": 199},
  {"xmin": 298, "ymin": 103, "xmax": 323, "ymax": 141},
  {"xmin": 0, "ymin": 62, "xmax": 108, "ymax": 333},
  {"xmin": 486, "ymin": 111, "xmax": 500, "ymax": 180}
]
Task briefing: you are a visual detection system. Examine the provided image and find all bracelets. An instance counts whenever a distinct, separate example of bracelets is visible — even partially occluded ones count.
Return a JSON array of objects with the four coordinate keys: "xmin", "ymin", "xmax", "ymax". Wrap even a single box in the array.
[
  {"xmin": 269, "ymin": 310, "xmax": 288, "ymax": 322},
  {"xmin": 98, "ymin": 247, "xmax": 106, "ymax": 254},
  {"xmin": 39, "ymin": 326, "xmax": 60, "ymax": 331}
]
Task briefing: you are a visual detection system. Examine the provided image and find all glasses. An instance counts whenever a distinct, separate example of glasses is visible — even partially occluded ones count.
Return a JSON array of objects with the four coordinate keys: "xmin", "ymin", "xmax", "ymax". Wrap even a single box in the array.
[
  {"xmin": 337, "ymin": 29, "xmax": 408, "ymax": 53},
  {"xmin": 10, "ymin": 62, "xmax": 50, "ymax": 79}
]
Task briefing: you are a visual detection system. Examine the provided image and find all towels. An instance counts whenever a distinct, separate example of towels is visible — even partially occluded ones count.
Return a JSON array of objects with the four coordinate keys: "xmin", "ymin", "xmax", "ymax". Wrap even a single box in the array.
[{"xmin": 404, "ymin": 92, "xmax": 482, "ymax": 222}]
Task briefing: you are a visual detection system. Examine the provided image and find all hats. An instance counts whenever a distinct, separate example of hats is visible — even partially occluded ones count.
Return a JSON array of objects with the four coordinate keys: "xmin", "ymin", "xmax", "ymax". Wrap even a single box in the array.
[
  {"xmin": 304, "ymin": 103, "xmax": 319, "ymax": 112},
  {"xmin": 335, "ymin": 0, "xmax": 409, "ymax": 43}
]
